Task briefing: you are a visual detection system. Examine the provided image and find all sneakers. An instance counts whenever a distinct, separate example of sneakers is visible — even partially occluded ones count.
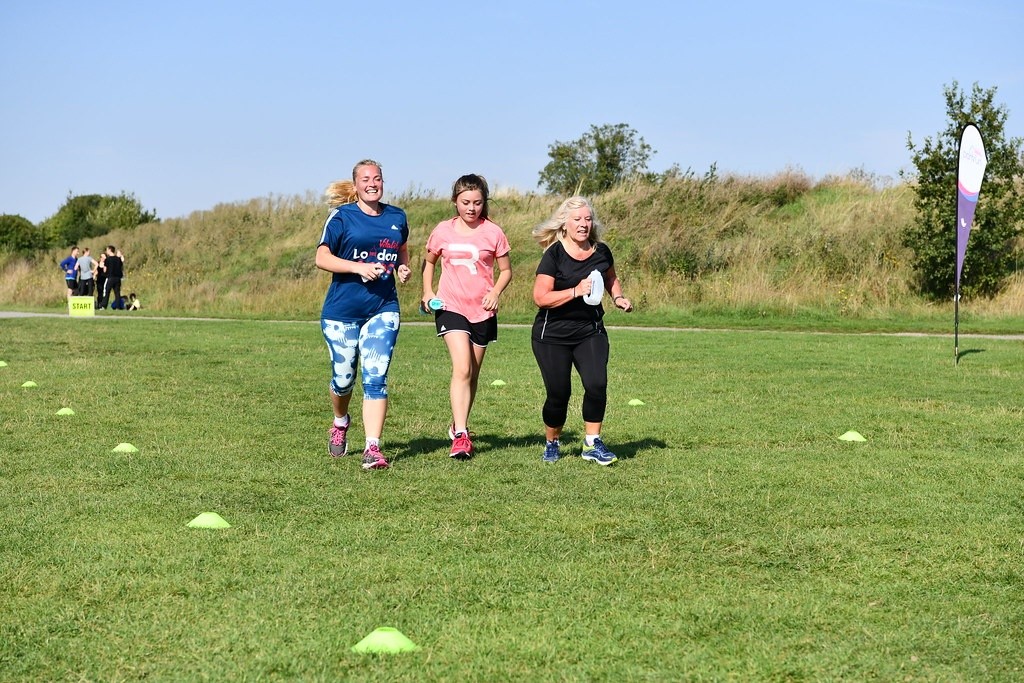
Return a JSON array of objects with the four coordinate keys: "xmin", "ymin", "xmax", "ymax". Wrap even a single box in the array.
[
  {"xmin": 328, "ymin": 413, "xmax": 350, "ymax": 458},
  {"xmin": 449, "ymin": 432, "xmax": 472, "ymax": 459},
  {"xmin": 581, "ymin": 438, "xmax": 618, "ymax": 466},
  {"xmin": 543, "ymin": 438, "xmax": 560, "ymax": 463},
  {"xmin": 448, "ymin": 421, "xmax": 470, "ymax": 440},
  {"xmin": 361, "ymin": 444, "xmax": 389, "ymax": 470}
]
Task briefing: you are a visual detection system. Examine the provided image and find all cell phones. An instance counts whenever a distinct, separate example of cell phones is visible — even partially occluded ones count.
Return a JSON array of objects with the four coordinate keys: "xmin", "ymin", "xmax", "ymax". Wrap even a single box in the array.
[{"xmin": 361, "ymin": 261, "xmax": 383, "ymax": 283}]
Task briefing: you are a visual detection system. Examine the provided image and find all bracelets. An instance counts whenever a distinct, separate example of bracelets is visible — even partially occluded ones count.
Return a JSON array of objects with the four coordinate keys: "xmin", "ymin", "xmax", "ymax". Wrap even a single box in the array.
[
  {"xmin": 574, "ymin": 286, "xmax": 575, "ymax": 298},
  {"xmin": 613, "ymin": 295, "xmax": 625, "ymax": 303}
]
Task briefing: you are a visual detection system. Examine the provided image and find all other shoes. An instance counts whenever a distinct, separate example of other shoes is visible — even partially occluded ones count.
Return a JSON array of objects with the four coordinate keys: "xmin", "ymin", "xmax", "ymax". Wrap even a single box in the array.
[{"xmin": 99, "ymin": 306, "xmax": 106, "ymax": 310}]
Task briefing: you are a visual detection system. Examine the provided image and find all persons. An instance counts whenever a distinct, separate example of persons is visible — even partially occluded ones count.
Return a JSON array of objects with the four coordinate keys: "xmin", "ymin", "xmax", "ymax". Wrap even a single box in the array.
[
  {"xmin": 420, "ymin": 174, "xmax": 512, "ymax": 458},
  {"xmin": 530, "ymin": 195, "xmax": 633, "ymax": 467},
  {"xmin": 314, "ymin": 159, "xmax": 412, "ymax": 470},
  {"xmin": 59, "ymin": 245, "xmax": 142, "ymax": 311}
]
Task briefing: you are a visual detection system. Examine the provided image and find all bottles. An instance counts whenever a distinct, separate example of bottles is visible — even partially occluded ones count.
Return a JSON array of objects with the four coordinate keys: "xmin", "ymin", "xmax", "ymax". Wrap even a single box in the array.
[
  {"xmin": 583, "ymin": 269, "xmax": 604, "ymax": 305},
  {"xmin": 419, "ymin": 299, "xmax": 443, "ymax": 315}
]
